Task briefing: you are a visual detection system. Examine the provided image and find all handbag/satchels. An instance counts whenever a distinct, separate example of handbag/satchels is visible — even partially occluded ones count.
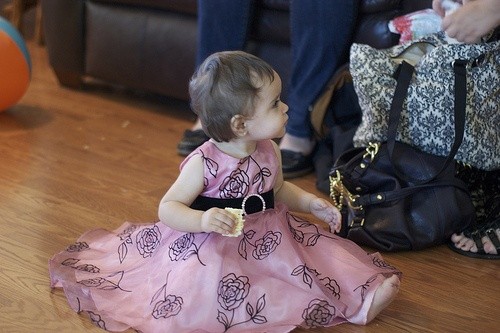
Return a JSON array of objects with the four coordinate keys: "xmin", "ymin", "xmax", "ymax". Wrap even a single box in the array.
[
  {"xmin": 329, "ymin": 139, "xmax": 479, "ymax": 251},
  {"xmin": 350, "ymin": 32, "xmax": 500, "ymax": 170}
]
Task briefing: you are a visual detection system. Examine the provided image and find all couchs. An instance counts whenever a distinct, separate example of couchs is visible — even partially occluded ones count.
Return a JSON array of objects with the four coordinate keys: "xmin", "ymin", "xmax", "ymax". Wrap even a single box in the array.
[{"xmin": 41, "ymin": 0, "xmax": 433, "ymax": 102}]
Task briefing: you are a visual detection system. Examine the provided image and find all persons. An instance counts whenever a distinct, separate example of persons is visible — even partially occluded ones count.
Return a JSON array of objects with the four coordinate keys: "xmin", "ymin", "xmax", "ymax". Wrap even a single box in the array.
[
  {"xmin": 177, "ymin": 0, "xmax": 360, "ymax": 180},
  {"xmin": 432, "ymin": 0, "xmax": 500, "ymax": 259},
  {"xmin": 48, "ymin": 49, "xmax": 403, "ymax": 333}
]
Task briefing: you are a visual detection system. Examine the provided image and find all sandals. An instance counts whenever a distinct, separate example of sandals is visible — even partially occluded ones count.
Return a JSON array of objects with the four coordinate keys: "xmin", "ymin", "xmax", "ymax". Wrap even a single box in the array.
[{"xmin": 448, "ymin": 227, "xmax": 500, "ymax": 259}]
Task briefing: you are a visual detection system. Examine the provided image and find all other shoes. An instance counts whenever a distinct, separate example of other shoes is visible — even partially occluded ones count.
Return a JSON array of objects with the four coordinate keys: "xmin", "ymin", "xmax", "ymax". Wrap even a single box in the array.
[
  {"xmin": 177, "ymin": 129, "xmax": 210, "ymax": 154},
  {"xmin": 278, "ymin": 134, "xmax": 323, "ymax": 178}
]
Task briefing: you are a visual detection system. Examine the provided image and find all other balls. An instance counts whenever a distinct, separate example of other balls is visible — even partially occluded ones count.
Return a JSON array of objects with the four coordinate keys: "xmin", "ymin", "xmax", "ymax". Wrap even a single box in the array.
[{"xmin": 0, "ymin": 15, "xmax": 32, "ymax": 113}]
